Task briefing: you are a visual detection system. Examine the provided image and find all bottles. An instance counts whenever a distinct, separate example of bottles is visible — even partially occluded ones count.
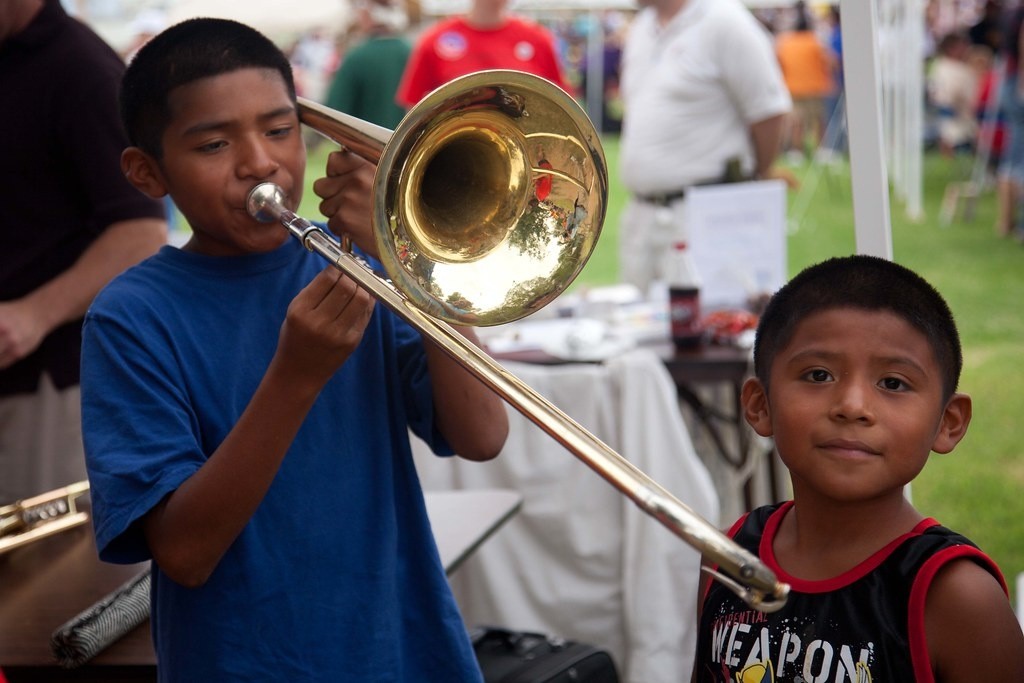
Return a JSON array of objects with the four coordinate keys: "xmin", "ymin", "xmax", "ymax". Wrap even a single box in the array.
[{"xmin": 667, "ymin": 240, "xmax": 702, "ymax": 351}]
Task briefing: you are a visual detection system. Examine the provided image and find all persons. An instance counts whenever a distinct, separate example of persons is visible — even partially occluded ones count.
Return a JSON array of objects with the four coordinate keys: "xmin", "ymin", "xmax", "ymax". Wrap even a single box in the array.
[
  {"xmin": 79, "ymin": 17, "xmax": 510, "ymax": 683},
  {"xmin": 0, "ymin": 0, "xmax": 170, "ymax": 503},
  {"xmin": 397, "ymin": 1, "xmax": 565, "ymax": 111},
  {"xmin": 777, "ymin": 1, "xmax": 1024, "ymax": 233},
  {"xmin": 691, "ymin": 255, "xmax": 1024, "ymax": 683},
  {"xmin": 616, "ymin": 0, "xmax": 793, "ymax": 299},
  {"xmin": 321, "ymin": 1, "xmax": 411, "ymax": 131}
]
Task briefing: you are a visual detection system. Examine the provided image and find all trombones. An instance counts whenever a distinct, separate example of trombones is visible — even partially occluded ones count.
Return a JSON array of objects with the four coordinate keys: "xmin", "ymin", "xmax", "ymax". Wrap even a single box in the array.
[{"xmin": 245, "ymin": 70, "xmax": 807, "ymax": 614}]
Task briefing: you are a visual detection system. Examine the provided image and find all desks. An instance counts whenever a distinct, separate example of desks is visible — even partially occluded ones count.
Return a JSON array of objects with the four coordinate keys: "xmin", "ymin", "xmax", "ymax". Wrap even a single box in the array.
[
  {"xmin": 389, "ymin": 284, "xmax": 782, "ymax": 683},
  {"xmin": 0, "ymin": 490, "xmax": 524, "ymax": 682}
]
